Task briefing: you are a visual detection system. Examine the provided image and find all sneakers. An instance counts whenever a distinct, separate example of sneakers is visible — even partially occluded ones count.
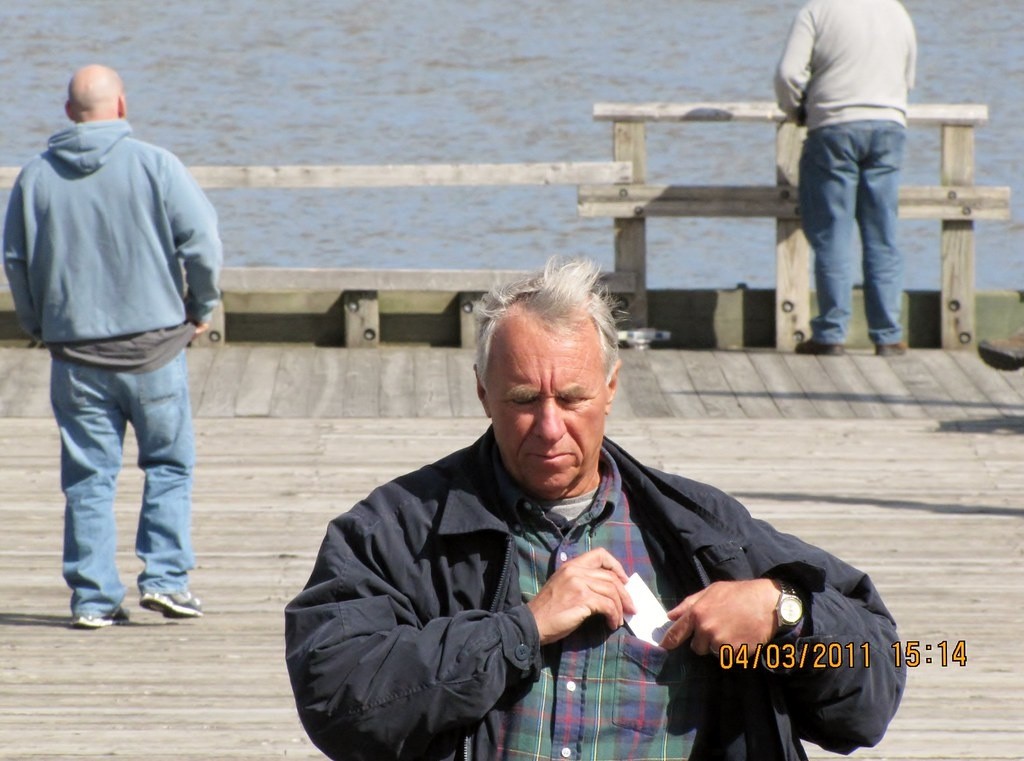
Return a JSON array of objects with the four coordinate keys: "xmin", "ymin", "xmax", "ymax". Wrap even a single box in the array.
[
  {"xmin": 69, "ymin": 604, "xmax": 130, "ymax": 628},
  {"xmin": 139, "ymin": 588, "xmax": 203, "ymax": 617}
]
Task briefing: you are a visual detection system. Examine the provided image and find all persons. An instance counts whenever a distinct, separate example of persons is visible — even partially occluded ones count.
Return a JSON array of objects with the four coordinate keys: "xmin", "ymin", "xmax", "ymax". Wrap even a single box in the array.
[
  {"xmin": 775, "ymin": 0, "xmax": 917, "ymax": 356},
  {"xmin": 286, "ymin": 262, "xmax": 906, "ymax": 761},
  {"xmin": 5, "ymin": 65, "xmax": 221, "ymax": 626}
]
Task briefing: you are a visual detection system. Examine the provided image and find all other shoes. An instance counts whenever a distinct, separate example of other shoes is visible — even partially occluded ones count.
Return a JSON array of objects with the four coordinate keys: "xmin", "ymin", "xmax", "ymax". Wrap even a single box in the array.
[
  {"xmin": 796, "ymin": 339, "xmax": 845, "ymax": 356},
  {"xmin": 876, "ymin": 343, "xmax": 906, "ymax": 355}
]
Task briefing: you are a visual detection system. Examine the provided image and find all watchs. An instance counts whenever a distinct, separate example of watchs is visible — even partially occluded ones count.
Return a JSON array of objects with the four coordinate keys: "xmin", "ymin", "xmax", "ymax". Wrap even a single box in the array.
[{"xmin": 774, "ymin": 578, "xmax": 804, "ymax": 635}]
